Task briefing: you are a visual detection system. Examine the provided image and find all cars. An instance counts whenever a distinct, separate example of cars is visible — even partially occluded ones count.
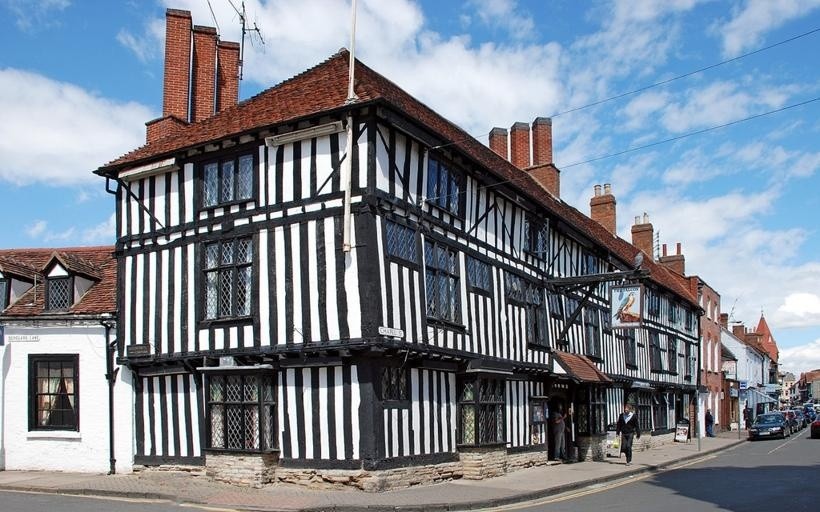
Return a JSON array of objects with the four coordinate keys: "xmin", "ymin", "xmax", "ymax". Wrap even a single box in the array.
[{"xmin": 748, "ymin": 402, "xmax": 816, "ymax": 440}]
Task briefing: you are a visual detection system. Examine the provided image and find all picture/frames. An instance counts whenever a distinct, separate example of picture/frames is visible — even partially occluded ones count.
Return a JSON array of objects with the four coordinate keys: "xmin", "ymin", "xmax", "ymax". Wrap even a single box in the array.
[{"xmin": 609, "ymin": 284, "xmax": 645, "ymax": 329}]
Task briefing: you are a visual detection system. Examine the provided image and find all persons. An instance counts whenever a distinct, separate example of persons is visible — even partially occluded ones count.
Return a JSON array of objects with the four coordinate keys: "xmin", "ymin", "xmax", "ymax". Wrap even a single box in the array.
[
  {"xmin": 616, "ymin": 403, "xmax": 641, "ymax": 466},
  {"xmin": 550, "ymin": 402, "xmax": 568, "ymax": 460},
  {"xmin": 708, "ymin": 408, "xmax": 713, "ymax": 436}
]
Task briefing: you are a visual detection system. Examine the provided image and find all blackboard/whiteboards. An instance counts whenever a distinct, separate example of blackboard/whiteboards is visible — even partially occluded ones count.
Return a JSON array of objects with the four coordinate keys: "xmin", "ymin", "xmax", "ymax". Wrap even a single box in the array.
[
  {"xmin": 606, "ymin": 431, "xmax": 622, "ymax": 458},
  {"xmin": 676, "ymin": 424, "xmax": 689, "ymax": 443}
]
